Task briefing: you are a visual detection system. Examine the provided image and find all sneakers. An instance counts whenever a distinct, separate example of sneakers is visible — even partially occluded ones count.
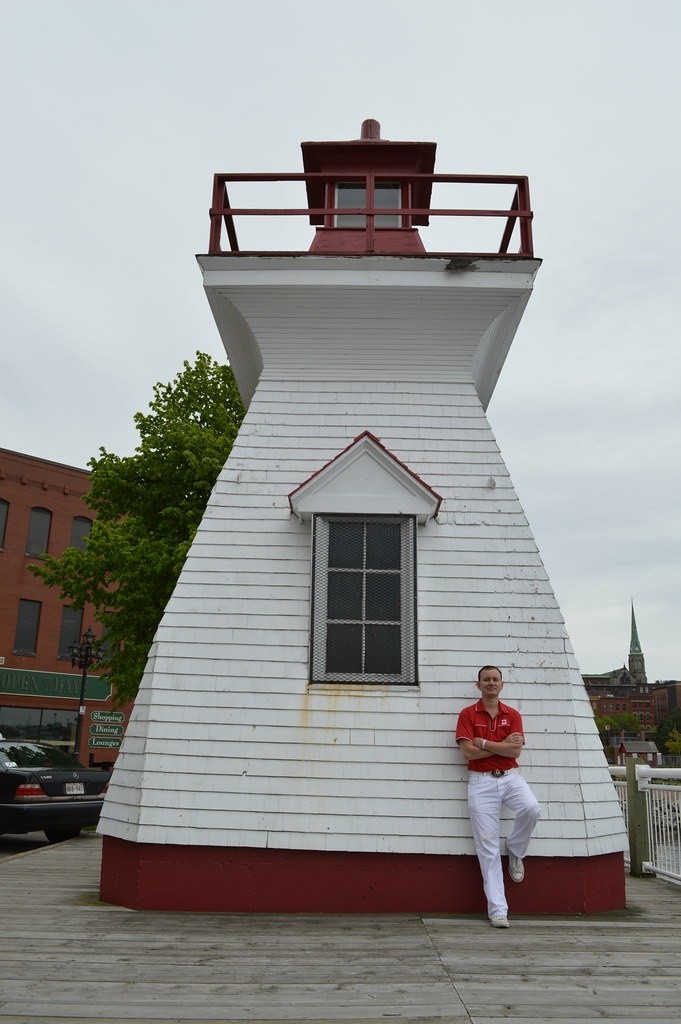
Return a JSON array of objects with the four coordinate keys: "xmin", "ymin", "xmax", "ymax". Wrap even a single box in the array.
[
  {"xmin": 491, "ymin": 916, "xmax": 509, "ymax": 928},
  {"xmin": 508, "ymin": 847, "xmax": 524, "ymax": 882}
]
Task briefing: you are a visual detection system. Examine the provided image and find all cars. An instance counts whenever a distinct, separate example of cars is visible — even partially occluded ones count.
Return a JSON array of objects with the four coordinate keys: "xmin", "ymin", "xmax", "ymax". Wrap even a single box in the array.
[{"xmin": 0, "ymin": 740, "xmax": 112, "ymax": 842}]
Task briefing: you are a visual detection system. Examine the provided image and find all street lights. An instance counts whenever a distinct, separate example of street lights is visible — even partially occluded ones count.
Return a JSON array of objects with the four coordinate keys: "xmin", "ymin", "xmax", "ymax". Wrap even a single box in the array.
[{"xmin": 68, "ymin": 625, "xmax": 106, "ymax": 764}]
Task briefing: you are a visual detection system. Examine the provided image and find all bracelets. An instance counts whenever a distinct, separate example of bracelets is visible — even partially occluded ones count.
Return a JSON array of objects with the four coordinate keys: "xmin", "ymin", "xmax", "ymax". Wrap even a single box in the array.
[{"xmin": 481, "ymin": 740, "xmax": 486, "ymax": 749}]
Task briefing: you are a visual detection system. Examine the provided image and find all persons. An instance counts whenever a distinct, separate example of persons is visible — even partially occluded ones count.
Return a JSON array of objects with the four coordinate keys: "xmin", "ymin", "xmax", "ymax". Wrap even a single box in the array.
[{"xmin": 456, "ymin": 665, "xmax": 541, "ymax": 928}]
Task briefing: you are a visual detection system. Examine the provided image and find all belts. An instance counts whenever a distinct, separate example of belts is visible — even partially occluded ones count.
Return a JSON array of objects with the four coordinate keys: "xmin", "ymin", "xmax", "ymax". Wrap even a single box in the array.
[{"xmin": 468, "ymin": 768, "xmax": 519, "ymax": 778}]
